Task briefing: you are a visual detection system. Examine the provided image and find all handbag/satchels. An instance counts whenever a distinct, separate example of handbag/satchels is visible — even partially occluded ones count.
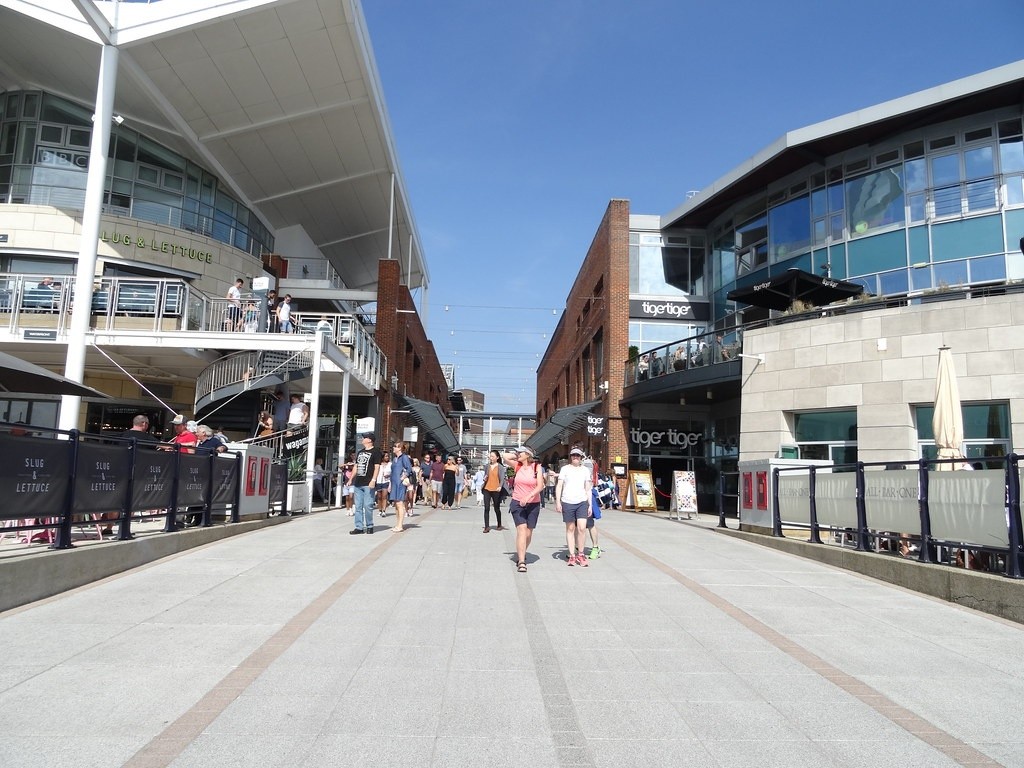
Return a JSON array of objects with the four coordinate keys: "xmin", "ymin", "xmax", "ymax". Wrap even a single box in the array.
[{"xmin": 400, "ymin": 454, "xmax": 410, "ymax": 486}]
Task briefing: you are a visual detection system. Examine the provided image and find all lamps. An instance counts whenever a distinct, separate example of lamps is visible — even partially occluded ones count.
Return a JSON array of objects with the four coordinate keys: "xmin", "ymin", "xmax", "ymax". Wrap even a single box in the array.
[
  {"xmin": 457, "ymin": 365, "xmax": 460, "ymax": 369},
  {"xmin": 680, "ymin": 398, "xmax": 685, "ymax": 405},
  {"xmin": 450, "ymin": 330, "xmax": 454, "ymax": 336},
  {"xmin": 543, "ymin": 333, "xmax": 547, "ymax": 339},
  {"xmin": 707, "ymin": 392, "xmax": 712, "ymax": 399},
  {"xmin": 113, "ymin": 115, "xmax": 124, "ymax": 126},
  {"xmin": 530, "ymin": 368, "xmax": 534, "ymax": 372},
  {"xmin": 819, "ymin": 262, "xmax": 832, "ymax": 276},
  {"xmin": 459, "ymin": 377, "xmax": 467, "ymax": 400},
  {"xmin": 536, "ymin": 353, "xmax": 539, "ymax": 358},
  {"xmin": 445, "ymin": 305, "xmax": 448, "ymax": 311},
  {"xmin": 552, "ymin": 309, "xmax": 557, "ymax": 315},
  {"xmin": 515, "ymin": 379, "xmax": 528, "ymax": 408},
  {"xmin": 454, "ymin": 351, "xmax": 457, "ymax": 355}
]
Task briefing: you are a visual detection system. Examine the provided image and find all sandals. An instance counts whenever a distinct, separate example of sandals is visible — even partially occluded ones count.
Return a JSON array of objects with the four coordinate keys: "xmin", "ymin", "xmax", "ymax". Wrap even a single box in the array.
[{"xmin": 518, "ymin": 561, "xmax": 527, "ymax": 572}]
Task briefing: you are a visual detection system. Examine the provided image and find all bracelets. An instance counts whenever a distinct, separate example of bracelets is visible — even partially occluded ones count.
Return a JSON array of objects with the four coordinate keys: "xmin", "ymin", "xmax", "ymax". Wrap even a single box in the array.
[{"xmin": 530, "ymin": 492, "xmax": 534, "ymax": 498}]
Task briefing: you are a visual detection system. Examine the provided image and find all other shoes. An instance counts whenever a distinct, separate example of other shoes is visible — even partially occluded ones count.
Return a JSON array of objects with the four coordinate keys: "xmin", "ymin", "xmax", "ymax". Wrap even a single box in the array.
[
  {"xmin": 350, "ymin": 512, "xmax": 353, "ymax": 516},
  {"xmin": 483, "ymin": 527, "xmax": 489, "ymax": 533},
  {"xmin": 391, "ymin": 527, "xmax": 404, "ymax": 531},
  {"xmin": 496, "ymin": 526, "xmax": 501, "ymax": 530},
  {"xmin": 404, "ymin": 499, "xmax": 485, "ymax": 516},
  {"xmin": 379, "ymin": 512, "xmax": 382, "ymax": 515},
  {"xmin": 350, "ymin": 529, "xmax": 363, "ymax": 534},
  {"xmin": 346, "ymin": 513, "xmax": 349, "ymax": 516},
  {"xmin": 324, "ymin": 499, "xmax": 328, "ymax": 504},
  {"xmin": 102, "ymin": 530, "xmax": 112, "ymax": 534},
  {"xmin": 367, "ymin": 528, "xmax": 373, "ymax": 534},
  {"xmin": 382, "ymin": 512, "xmax": 386, "ymax": 516}
]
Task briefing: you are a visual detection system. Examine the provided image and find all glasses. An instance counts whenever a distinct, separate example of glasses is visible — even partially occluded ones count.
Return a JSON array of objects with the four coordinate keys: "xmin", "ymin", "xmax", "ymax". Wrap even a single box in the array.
[{"xmin": 572, "ymin": 454, "xmax": 581, "ymax": 457}]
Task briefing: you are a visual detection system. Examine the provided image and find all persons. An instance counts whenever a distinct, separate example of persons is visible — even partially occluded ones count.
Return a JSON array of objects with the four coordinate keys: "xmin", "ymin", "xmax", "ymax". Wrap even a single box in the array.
[
  {"xmin": 556, "ymin": 446, "xmax": 592, "ymax": 567},
  {"xmin": 565, "ymin": 488, "xmax": 600, "ymax": 560},
  {"xmin": 226, "ymin": 278, "xmax": 356, "ymax": 342},
  {"xmin": 636, "ymin": 332, "xmax": 729, "ymax": 381},
  {"xmin": 502, "ymin": 446, "xmax": 544, "ymax": 572},
  {"xmin": 103, "ymin": 384, "xmax": 621, "ymax": 538},
  {"xmin": 36, "ymin": 277, "xmax": 145, "ymax": 317}
]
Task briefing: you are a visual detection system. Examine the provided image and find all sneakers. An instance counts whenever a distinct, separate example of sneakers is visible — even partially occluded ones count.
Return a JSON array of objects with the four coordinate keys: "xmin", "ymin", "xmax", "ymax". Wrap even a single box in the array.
[
  {"xmin": 589, "ymin": 547, "xmax": 601, "ymax": 559},
  {"xmin": 568, "ymin": 556, "xmax": 575, "ymax": 566},
  {"xmin": 576, "ymin": 555, "xmax": 588, "ymax": 567},
  {"xmin": 566, "ymin": 547, "xmax": 578, "ymax": 558}
]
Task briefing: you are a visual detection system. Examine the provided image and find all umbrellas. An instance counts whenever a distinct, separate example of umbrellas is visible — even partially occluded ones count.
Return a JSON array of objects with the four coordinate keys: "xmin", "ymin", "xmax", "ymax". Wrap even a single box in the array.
[
  {"xmin": 727, "ymin": 269, "xmax": 864, "ymax": 312},
  {"xmin": 0, "ymin": 352, "xmax": 111, "ymax": 398},
  {"xmin": 932, "ymin": 345, "xmax": 973, "ymax": 471}
]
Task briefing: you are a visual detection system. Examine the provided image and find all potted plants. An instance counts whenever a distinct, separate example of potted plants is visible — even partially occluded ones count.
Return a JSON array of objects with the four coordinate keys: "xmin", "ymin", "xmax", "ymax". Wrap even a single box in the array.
[
  {"xmin": 920, "ymin": 274, "xmax": 971, "ymax": 304},
  {"xmin": 845, "ymin": 293, "xmax": 887, "ymax": 314},
  {"xmin": 773, "ymin": 302, "xmax": 822, "ymax": 325},
  {"xmin": 1004, "ymin": 278, "xmax": 1024, "ymax": 295},
  {"xmin": 272, "ymin": 448, "xmax": 310, "ymax": 516}
]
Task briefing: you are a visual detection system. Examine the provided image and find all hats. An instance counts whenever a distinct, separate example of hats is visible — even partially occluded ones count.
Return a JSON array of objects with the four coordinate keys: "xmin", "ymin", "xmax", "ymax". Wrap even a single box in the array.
[
  {"xmin": 514, "ymin": 446, "xmax": 534, "ymax": 458},
  {"xmin": 570, "ymin": 449, "xmax": 584, "ymax": 456},
  {"xmin": 169, "ymin": 415, "xmax": 188, "ymax": 424},
  {"xmin": 362, "ymin": 432, "xmax": 376, "ymax": 442}
]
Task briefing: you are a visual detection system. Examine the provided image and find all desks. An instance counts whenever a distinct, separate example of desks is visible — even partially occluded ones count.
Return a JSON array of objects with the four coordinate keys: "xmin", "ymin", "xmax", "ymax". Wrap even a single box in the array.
[{"xmin": 673, "ymin": 359, "xmax": 686, "ymax": 372}]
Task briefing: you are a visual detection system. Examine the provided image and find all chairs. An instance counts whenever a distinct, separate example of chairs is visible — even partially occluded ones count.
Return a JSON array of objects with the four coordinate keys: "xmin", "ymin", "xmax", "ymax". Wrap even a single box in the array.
[
  {"xmin": 319, "ymin": 323, "xmax": 331, "ymax": 339},
  {"xmin": 652, "ymin": 335, "xmax": 742, "ymax": 376},
  {"xmin": 0, "ymin": 287, "xmax": 184, "ymax": 317}
]
